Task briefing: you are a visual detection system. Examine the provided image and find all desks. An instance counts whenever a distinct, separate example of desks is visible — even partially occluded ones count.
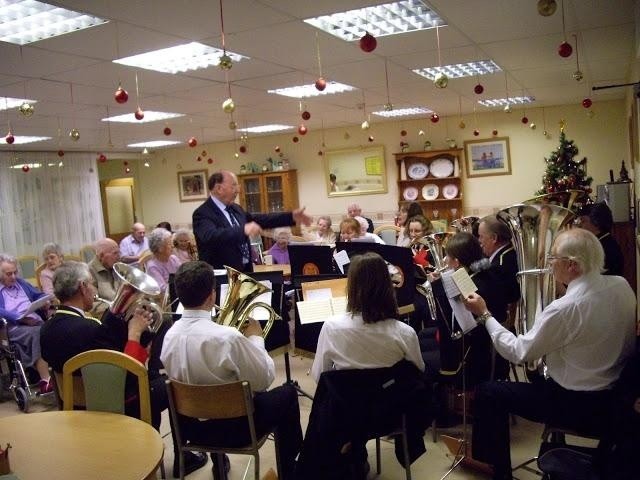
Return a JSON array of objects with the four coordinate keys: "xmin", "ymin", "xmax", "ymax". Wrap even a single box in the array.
[
  {"xmin": 610, "ymin": 219, "xmax": 636, "ymax": 299},
  {"xmin": 251, "ymin": 263, "xmax": 293, "ymax": 279},
  {"xmin": 0, "ymin": 407, "xmax": 165, "ymax": 480}
]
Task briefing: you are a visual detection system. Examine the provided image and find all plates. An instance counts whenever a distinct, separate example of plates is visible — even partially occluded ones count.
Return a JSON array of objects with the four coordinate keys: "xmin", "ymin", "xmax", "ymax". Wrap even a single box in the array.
[
  {"xmin": 402, "ymin": 186, "xmax": 418, "ymax": 201},
  {"xmin": 408, "ymin": 162, "xmax": 429, "ymax": 179},
  {"xmin": 442, "ymin": 183, "xmax": 458, "ymax": 199},
  {"xmin": 429, "ymin": 158, "xmax": 453, "ymax": 178},
  {"xmin": 421, "ymin": 183, "xmax": 439, "ymax": 200}
]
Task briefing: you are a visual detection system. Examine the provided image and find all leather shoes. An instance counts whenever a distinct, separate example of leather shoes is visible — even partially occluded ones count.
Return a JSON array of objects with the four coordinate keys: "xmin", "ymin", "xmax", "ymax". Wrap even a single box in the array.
[
  {"xmin": 212, "ymin": 453, "xmax": 230, "ymax": 480},
  {"xmin": 171, "ymin": 451, "xmax": 208, "ymax": 479}
]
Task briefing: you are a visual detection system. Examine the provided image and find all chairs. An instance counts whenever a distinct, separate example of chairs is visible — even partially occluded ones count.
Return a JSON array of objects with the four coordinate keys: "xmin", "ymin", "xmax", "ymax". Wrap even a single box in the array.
[
  {"xmin": 14, "ymin": 237, "xmax": 155, "ymax": 294},
  {"xmin": 373, "ymin": 224, "xmax": 401, "ymax": 247}
]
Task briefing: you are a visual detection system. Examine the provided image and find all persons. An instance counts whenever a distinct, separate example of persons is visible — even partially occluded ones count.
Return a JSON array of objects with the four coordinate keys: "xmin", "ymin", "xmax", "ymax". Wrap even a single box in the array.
[
  {"xmin": 310, "ymin": 200, "xmax": 637, "ymax": 480},
  {"xmin": 0, "ymin": 221, "xmax": 305, "ymax": 479},
  {"xmin": 191, "ymin": 170, "xmax": 313, "ymax": 275}
]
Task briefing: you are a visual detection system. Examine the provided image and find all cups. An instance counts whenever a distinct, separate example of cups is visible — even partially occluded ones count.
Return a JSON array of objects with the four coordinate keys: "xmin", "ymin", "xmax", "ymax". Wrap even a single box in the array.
[
  {"xmin": 266, "ymin": 178, "xmax": 283, "ymax": 213},
  {"xmin": 245, "ymin": 180, "xmax": 259, "ymax": 212}
]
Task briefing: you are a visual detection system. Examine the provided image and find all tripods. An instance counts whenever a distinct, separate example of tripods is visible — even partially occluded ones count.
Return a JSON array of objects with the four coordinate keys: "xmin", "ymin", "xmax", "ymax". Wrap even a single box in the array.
[
  {"xmin": 439, "ymin": 331, "xmax": 471, "ymax": 480},
  {"xmin": 282, "ymin": 350, "xmax": 315, "ymax": 403}
]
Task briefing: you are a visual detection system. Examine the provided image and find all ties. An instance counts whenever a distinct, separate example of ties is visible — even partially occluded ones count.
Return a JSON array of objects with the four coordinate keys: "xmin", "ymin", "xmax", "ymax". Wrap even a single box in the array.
[{"xmin": 225, "ymin": 206, "xmax": 250, "ymax": 260}]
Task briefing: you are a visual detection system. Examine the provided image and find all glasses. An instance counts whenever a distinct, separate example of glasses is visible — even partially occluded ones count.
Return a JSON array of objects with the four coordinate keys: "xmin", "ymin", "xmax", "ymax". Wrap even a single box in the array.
[{"xmin": 545, "ymin": 253, "xmax": 571, "ymax": 263}]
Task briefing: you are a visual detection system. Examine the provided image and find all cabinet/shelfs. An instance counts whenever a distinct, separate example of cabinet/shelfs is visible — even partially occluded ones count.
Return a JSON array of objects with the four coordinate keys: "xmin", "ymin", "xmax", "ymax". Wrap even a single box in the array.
[
  {"xmin": 232, "ymin": 167, "xmax": 303, "ymax": 251},
  {"xmin": 387, "ymin": 146, "xmax": 464, "ymax": 232}
]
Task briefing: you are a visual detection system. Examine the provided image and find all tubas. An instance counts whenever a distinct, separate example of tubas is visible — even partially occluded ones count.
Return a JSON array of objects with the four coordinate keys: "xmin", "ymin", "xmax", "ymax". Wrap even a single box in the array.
[
  {"xmin": 214, "ymin": 265, "xmax": 276, "ymax": 339},
  {"xmin": 495, "ymin": 189, "xmax": 586, "ymax": 383},
  {"xmin": 94, "ymin": 261, "xmax": 163, "ymax": 347},
  {"xmin": 414, "ymin": 230, "xmax": 449, "ymax": 320}
]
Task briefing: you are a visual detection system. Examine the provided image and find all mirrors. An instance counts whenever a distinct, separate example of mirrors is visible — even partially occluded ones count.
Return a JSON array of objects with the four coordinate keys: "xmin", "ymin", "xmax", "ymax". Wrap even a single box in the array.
[{"xmin": 319, "ymin": 142, "xmax": 389, "ymax": 199}]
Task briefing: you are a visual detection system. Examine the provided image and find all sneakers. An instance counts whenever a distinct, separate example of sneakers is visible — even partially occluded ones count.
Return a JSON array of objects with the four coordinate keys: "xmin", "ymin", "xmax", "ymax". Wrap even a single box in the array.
[{"xmin": 37, "ymin": 374, "xmax": 58, "ymax": 397}]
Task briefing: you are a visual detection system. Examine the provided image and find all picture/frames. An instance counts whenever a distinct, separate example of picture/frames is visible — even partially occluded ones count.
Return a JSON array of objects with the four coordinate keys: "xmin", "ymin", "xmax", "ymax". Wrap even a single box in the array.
[
  {"xmin": 462, "ymin": 135, "xmax": 513, "ymax": 180},
  {"xmin": 176, "ymin": 168, "xmax": 209, "ymax": 203}
]
